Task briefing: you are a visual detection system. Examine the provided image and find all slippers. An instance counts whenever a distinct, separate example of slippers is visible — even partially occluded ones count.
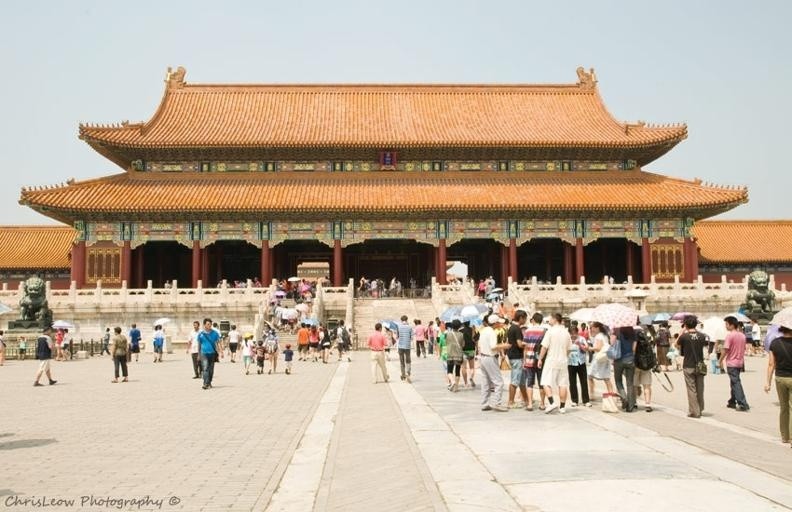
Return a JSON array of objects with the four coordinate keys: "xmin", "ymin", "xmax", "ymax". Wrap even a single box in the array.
[{"xmin": 635, "ymin": 328, "xmax": 657, "ymax": 370}]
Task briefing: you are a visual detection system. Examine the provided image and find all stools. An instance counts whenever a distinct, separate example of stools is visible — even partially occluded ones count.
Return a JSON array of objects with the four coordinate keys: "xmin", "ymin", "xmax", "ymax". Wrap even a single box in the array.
[{"xmin": 480, "ymin": 353, "xmax": 490, "ymax": 357}]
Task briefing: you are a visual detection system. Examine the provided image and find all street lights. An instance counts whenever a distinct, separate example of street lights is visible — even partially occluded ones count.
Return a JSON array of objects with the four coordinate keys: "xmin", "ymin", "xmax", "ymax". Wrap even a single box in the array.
[
  {"xmin": 607, "ymin": 339, "xmax": 622, "ymax": 361},
  {"xmin": 602, "ymin": 392, "xmax": 621, "ymax": 413},
  {"xmin": 696, "ymin": 361, "xmax": 708, "ymax": 376}
]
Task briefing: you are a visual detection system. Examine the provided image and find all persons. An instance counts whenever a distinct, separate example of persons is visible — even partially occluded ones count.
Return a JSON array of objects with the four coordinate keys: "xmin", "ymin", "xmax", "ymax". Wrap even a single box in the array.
[{"xmin": 0, "ymin": 255, "xmax": 792, "ymax": 450}]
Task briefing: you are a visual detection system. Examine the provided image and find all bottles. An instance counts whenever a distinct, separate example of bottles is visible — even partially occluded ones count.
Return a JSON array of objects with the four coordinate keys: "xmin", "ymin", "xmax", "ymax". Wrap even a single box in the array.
[{"xmin": 488, "ymin": 314, "xmax": 506, "ymax": 324}]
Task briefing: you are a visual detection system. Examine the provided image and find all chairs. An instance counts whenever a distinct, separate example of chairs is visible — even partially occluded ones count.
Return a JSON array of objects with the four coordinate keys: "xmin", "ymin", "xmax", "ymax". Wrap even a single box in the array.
[
  {"xmin": 727, "ymin": 402, "xmax": 746, "ymax": 410},
  {"xmin": 447, "ymin": 378, "xmax": 476, "ymax": 391},
  {"xmin": 622, "ymin": 399, "xmax": 653, "ymax": 412},
  {"xmin": 481, "ymin": 399, "xmax": 592, "ymax": 414}
]
